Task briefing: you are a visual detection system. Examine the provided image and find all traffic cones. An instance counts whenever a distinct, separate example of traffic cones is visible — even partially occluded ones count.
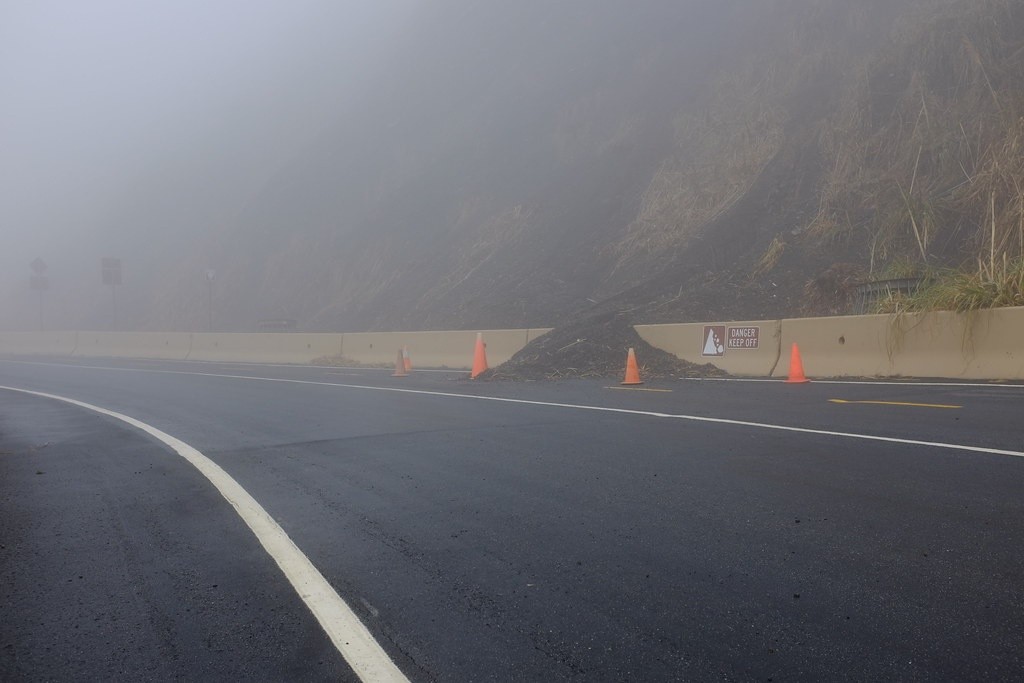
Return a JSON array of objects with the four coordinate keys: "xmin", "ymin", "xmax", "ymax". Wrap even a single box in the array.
[
  {"xmin": 468, "ymin": 332, "xmax": 488, "ymax": 380},
  {"xmin": 390, "ymin": 346, "xmax": 409, "ymax": 377},
  {"xmin": 401, "ymin": 345, "xmax": 413, "ymax": 372},
  {"xmin": 782, "ymin": 342, "xmax": 812, "ymax": 383},
  {"xmin": 621, "ymin": 347, "xmax": 645, "ymax": 385}
]
